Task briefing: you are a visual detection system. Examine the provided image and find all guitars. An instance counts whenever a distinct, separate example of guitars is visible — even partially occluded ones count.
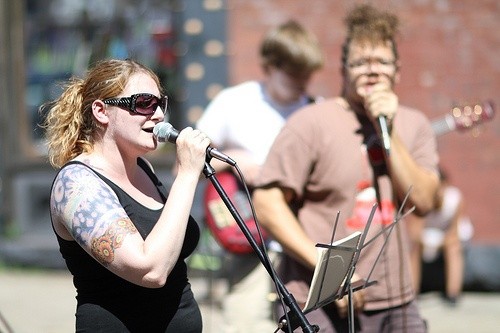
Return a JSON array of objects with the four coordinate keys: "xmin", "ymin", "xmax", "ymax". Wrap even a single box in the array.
[{"xmin": 202, "ymin": 99, "xmax": 497, "ymax": 256}]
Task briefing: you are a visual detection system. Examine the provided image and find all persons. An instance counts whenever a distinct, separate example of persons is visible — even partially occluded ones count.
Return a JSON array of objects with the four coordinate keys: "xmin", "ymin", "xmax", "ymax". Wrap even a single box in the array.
[
  {"xmin": 34, "ymin": 59, "xmax": 217, "ymax": 333},
  {"xmin": 252, "ymin": 3, "xmax": 440, "ymax": 333},
  {"xmin": 172, "ymin": 19, "xmax": 327, "ymax": 333}
]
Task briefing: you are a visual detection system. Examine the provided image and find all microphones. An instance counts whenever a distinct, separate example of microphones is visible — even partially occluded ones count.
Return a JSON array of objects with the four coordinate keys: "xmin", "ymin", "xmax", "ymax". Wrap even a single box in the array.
[
  {"xmin": 153, "ymin": 121, "xmax": 236, "ymax": 166},
  {"xmin": 377, "ymin": 115, "xmax": 392, "ymax": 152}
]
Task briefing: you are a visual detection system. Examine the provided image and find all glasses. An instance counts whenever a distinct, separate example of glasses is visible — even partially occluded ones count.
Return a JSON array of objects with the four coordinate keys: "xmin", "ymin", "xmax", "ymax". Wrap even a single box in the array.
[{"xmin": 103, "ymin": 93, "xmax": 168, "ymax": 116}]
